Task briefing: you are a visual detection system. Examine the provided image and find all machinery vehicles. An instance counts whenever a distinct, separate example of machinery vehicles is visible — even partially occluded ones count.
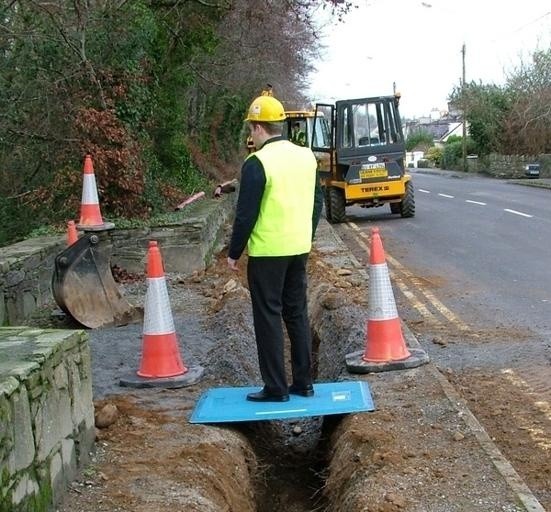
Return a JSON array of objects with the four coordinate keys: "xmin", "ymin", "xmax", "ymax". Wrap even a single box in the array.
[
  {"xmin": 244, "ymin": 82, "xmax": 333, "ymax": 199},
  {"xmin": 308, "ymin": 81, "xmax": 415, "ymax": 226}
]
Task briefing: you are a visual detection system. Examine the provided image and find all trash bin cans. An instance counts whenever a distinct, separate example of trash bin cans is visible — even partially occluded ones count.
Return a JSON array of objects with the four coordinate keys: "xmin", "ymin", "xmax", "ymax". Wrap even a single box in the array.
[{"xmin": 466, "ymin": 154, "xmax": 478, "ymax": 171}]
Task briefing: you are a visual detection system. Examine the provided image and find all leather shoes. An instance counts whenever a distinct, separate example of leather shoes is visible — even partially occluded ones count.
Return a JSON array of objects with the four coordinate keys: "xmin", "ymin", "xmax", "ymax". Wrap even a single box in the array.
[
  {"xmin": 288, "ymin": 384, "xmax": 316, "ymax": 397},
  {"xmin": 244, "ymin": 382, "xmax": 291, "ymax": 404}
]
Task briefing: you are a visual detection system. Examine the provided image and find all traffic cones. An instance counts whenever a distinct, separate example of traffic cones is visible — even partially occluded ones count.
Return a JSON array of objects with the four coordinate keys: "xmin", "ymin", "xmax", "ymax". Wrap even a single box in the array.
[
  {"xmin": 75, "ymin": 152, "xmax": 115, "ymax": 233},
  {"xmin": 342, "ymin": 224, "xmax": 431, "ymax": 376},
  {"xmin": 118, "ymin": 240, "xmax": 206, "ymax": 388},
  {"xmin": 67, "ymin": 219, "xmax": 79, "ymax": 250}
]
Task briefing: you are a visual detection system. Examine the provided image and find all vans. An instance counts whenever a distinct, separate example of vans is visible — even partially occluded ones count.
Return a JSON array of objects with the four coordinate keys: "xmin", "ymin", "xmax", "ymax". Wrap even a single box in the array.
[{"xmin": 524, "ymin": 163, "xmax": 540, "ymax": 176}]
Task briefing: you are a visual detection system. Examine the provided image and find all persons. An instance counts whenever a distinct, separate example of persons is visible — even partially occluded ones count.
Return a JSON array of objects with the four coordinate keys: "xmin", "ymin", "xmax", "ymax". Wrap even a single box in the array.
[
  {"xmin": 224, "ymin": 93, "xmax": 325, "ymax": 402},
  {"xmin": 291, "ymin": 123, "xmax": 307, "ymax": 147}
]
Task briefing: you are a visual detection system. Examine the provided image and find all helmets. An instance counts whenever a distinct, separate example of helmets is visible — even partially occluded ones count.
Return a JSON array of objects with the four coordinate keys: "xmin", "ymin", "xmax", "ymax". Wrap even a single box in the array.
[{"xmin": 241, "ymin": 95, "xmax": 288, "ymax": 125}]
